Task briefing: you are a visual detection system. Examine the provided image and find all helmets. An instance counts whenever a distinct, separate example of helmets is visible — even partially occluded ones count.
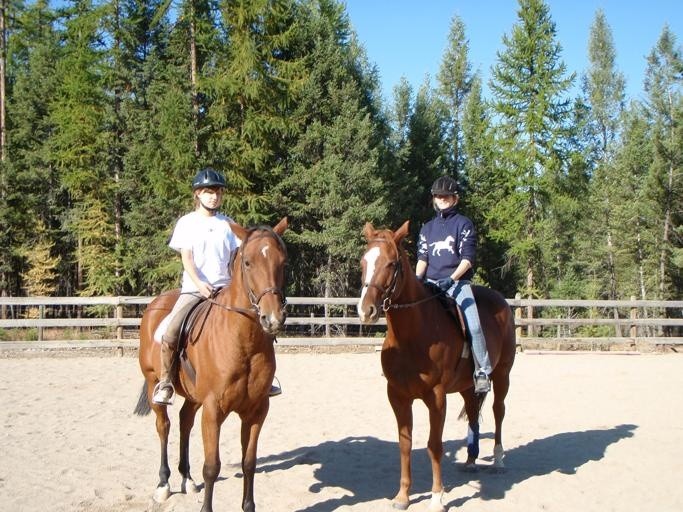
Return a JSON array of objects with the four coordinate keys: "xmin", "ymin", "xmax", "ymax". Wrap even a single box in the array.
[
  {"xmin": 431, "ymin": 176, "xmax": 458, "ymax": 196},
  {"xmin": 192, "ymin": 167, "xmax": 225, "ymax": 189}
]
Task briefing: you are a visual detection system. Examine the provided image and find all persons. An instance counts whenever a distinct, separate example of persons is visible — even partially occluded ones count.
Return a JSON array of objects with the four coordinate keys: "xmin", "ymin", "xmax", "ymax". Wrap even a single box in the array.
[
  {"xmin": 153, "ymin": 171, "xmax": 282, "ymax": 404},
  {"xmin": 414, "ymin": 175, "xmax": 492, "ymax": 393}
]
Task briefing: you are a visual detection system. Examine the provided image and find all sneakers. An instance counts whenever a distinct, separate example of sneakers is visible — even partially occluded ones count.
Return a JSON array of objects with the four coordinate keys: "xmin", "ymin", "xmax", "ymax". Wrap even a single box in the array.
[
  {"xmin": 269, "ymin": 385, "xmax": 279, "ymax": 394},
  {"xmin": 476, "ymin": 372, "xmax": 491, "ymax": 391},
  {"xmin": 153, "ymin": 387, "xmax": 173, "ymax": 404}
]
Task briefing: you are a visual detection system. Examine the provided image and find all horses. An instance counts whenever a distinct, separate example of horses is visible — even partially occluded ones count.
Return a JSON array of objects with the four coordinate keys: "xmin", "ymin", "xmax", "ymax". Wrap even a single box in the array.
[
  {"xmin": 355, "ymin": 219, "xmax": 517, "ymax": 512},
  {"xmin": 132, "ymin": 215, "xmax": 289, "ymax": 511}
]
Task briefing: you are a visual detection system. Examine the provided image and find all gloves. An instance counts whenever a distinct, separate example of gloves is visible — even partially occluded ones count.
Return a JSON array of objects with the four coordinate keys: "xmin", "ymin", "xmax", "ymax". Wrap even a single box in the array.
[{"xmin": 438, "ymin": 276, "xmax": 452, "ymax": 291}]
